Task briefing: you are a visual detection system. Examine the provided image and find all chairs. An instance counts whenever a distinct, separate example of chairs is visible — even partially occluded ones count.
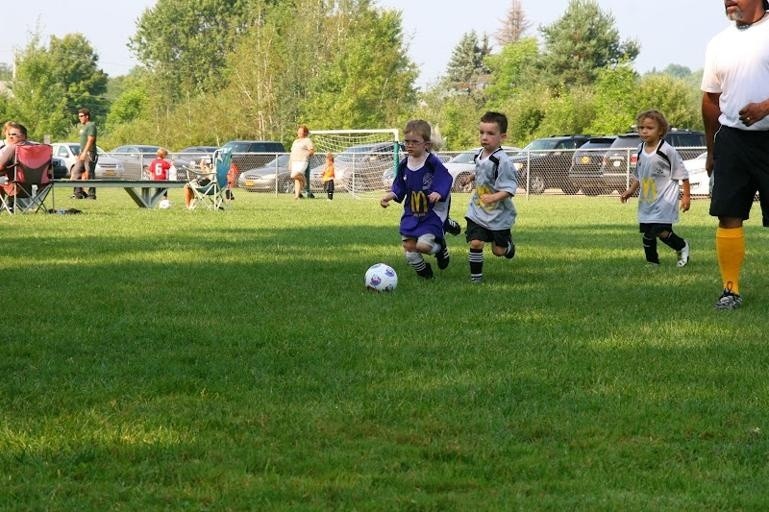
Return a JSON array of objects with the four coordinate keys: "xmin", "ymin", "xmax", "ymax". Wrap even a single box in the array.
[
  {"xmin": 0, "ymin": 143, "xmax": 55, "ymax": 216},
  {"xmin": 183, "ymin": 148, "xmax": 233, "ymax": 212}
]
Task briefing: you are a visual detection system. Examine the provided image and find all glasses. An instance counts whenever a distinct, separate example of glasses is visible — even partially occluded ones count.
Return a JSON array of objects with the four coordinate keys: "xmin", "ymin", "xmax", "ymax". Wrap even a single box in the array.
[
  {"xmin": 79, "ymin": 115, "xmax": 84, "ymax": 117},
  {"xmin": 8, "ymin": 134, "xmax": 17, "ymax": 135},
  {"xmin": 403, "ymin": 140, "xmax": 425, "ymax": 147}
]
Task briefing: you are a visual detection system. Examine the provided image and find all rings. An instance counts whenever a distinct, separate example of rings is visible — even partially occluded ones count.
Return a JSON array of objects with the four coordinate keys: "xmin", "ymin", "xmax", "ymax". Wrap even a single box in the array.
[{"xmin": 747, "ymin": 116, "xmax": 751, "ymax": 122}]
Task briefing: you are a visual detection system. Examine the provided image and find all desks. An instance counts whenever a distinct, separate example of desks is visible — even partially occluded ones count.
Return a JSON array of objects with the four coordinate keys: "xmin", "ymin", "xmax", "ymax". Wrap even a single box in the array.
[{"xmin": 43, "ymin": 179, "xmax": 190, "ymax": 209}]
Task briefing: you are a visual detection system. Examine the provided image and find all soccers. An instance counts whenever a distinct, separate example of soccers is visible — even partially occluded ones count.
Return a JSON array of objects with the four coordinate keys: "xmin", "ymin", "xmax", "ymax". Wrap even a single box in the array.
[{"xmin": 364, "ymin": 263, "xmax": 397, "ymax": 293}]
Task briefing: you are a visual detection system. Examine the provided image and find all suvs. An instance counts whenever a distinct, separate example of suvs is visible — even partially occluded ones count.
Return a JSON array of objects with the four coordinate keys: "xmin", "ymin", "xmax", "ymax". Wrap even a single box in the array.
[
  {"xmin": 509, "ymin": 135, "xmax": 588, "ymax": 195},
  {"xmin": 568, "ymin": 136, "xmax": 615, "ymax": 195},
  {"xmin": 601, "ymin": 132, "xmax": 705, "ymax": 195}
]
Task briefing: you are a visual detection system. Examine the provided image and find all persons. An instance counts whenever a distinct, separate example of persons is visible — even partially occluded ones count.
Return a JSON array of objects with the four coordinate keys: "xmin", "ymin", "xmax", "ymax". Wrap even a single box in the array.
[
  {"xmin": 150, "ymin": 148, "xmax": 172, "ymax": 199},
  {"xmin": 698, "ymin": 0, "xmax": 768, "ymax": 311},
  {"xmin": 0, "ymin": 124, "xmax": 35, "ymax": 213},
  {"xmin": 425, "ymin": 122, "xmax": 460, "ymax": 240},
  {"xmin": 619, "ymin": 110, "xmax": 691, "ymax": 269},
  {"xmin": 458, "ymin": 112, "xmax": 520, "ymax": 287},
  {"xmin": 226, "ymin": 156, "xmax": 239, "ymax": 200},
  {"xmin": 0, "ymin": 120, "xmax": 24, "ymax": 150},
  {"xmin": 320, "ymin": 153, "xmax": 336, "ymax": 202},
  {"xmin": 141, "ymin": 164, "xmax": 150, "ymax": 180},
  {"xmin": 69, "ymin": 108, "xmax": 99, "ymax": 199},
  {"xmin": 287, "ymin": 124, "xmax": 314, "ymax": 199},
  {"xmin": 183, "ymin": 159, "xmax": 220, "ymax": 210},
  {"xmin": 379, "ymin": 120, "xmax": 451, "ymax": 279}
]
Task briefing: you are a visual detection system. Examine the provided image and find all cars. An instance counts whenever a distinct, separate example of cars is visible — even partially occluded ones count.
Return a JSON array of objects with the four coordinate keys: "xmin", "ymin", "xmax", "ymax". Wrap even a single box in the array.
[
  {"xmin": 238, "ymin": 152, "xmax": 337, "ymax": 194},
  {"xmin": 1, "ymin": 140, "xmax": 68, "ymax": 178},
  {"xmin": 442, "ymin": 148, "xmax": 521, "ymax": 192},
  {"xmin": 310, "ymin": 143, "xmax": 405, "ymax": 191},
  {"xmin": 683, "ymin": 150, "xmax": 709, "ymax": 195},
  {"xmin": 177, "ymin": 146, "xmax": 217, "ymax": 163},
  {"xmin": 110, "ymin": 145, "xmax": 194, "ymax": 180},
  {"xmin": 52, "ymin": 142, "xmax": 124, "ymax": 180},
  {"xmin": 383, "ymin": 155, "xmax": 450, "ymax": 193},
  {"xmin": 224, "ymin": 141, "xmax": 284, "ymax": 173}
]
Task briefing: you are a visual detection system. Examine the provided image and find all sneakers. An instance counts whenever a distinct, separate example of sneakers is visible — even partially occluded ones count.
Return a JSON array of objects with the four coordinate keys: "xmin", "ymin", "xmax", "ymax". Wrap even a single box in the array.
[
  {"xmin": 676, "ymin": 238, "xmax": 689, "ymax": 267},
  {"xmin": 434, "ymin": 239, "xmax": 449, "ymax": 270},
  {"xmin": 417, "ymin": 263, "xmax": 433, "ymax": 278},
  {"xmin": 713, "ymin": 289, "xmax": 743, "ymax": 310},
  {"xmin": 444, "ymin": 217, "xmax": 461, "ymax": 235},
  {"xmin": 505, "ymin": 234, "xmax": 515, "ymax": 259}
]
